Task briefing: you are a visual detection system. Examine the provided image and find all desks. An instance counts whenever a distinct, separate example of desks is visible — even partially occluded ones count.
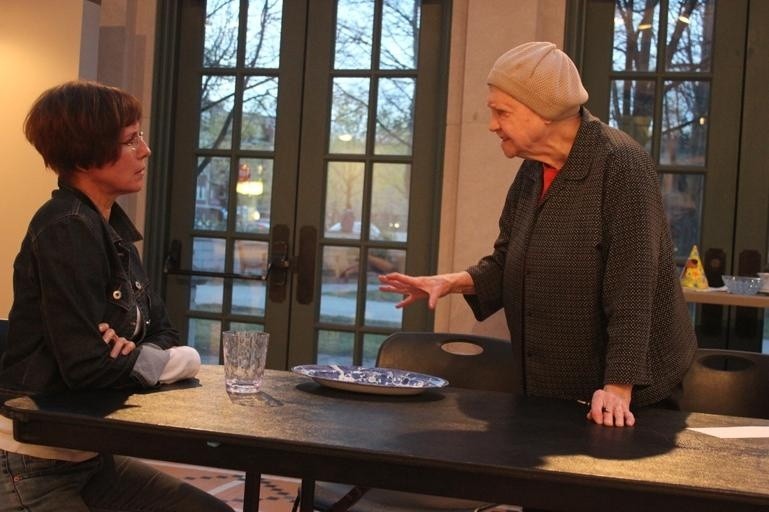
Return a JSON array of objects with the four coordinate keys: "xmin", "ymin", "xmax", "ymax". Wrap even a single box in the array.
[
  {"xmin": 1, "ymin": 363, "xmax": 768, "ymax": 512},
  {"xmin": 681, "ymin": 290, "xmax": 769, "ymax": 372}
]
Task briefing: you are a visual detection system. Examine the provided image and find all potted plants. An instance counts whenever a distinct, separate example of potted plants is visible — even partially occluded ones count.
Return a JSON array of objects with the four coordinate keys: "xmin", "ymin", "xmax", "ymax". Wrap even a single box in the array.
[{"xmin": 368, "ymin": 226, "xmax": 396, "ymax": 275}]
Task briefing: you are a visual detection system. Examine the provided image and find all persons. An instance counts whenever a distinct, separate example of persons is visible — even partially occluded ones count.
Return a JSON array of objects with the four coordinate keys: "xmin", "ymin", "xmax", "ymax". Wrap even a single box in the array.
[
  {"xmin": 323, "ymin": 210, "xmax": 359, "ymax": 281},
  {"xmin": 367, "ymin": 232, "xmax": 396, "ymax": 274},
  {"xmin": 0, "ymin": 77, "xmax": 238, "ymax": 511},
  {"xmin": 377, "ymin": 39, "xmax": 701, "ymax": 431}
]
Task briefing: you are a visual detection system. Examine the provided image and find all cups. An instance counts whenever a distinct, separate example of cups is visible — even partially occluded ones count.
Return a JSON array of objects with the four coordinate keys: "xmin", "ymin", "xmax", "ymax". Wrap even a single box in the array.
[{"xmin": 221, "ymin": 329, "xmax": 270, "ymax": 394}]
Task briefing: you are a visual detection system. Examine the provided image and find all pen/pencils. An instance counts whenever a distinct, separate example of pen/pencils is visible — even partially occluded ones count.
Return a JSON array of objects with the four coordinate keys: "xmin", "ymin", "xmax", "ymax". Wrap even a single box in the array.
[{"xmin": 577, "ymin": 400, "xmax": 610, "ymax": 412}]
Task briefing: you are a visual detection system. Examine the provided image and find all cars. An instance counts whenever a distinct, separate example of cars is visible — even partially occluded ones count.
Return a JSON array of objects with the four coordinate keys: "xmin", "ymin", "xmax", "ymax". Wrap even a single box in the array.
[{"xmin": 191, "ymin": 204, "xmax": 407, "ymax": 272}]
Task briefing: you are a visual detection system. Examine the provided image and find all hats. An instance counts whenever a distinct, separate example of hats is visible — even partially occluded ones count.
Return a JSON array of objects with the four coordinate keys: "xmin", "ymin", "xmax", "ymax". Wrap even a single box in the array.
[
  {"xmin": 679, "ymin": 245, "xmax": 709, "ymax": 291},
  {"xmin": 486, "ymin": 41, "xmax": 589, "ymax": 122}
]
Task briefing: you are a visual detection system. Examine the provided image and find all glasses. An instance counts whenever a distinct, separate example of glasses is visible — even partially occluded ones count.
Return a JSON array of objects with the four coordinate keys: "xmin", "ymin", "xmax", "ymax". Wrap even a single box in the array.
[{"xmin": 121, "ymin": 130, "xmax": 145, "ymax": 152}]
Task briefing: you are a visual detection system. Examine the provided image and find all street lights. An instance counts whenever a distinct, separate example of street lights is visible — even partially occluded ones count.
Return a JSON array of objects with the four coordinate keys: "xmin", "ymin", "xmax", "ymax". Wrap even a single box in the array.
[{"xmin": 336, "ymin": 131, "xmax": 356, "ymax": 234}]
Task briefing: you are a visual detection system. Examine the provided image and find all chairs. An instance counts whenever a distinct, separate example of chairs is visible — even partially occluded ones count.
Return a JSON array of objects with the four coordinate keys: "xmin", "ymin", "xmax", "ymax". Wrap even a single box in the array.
[
  {"xmin": 291, "ymin": 331, "xmax": 524, "ymax": 512},
  {"xmin": 677, "ymin": 349, "xmax": 769, "ymax": 421}
]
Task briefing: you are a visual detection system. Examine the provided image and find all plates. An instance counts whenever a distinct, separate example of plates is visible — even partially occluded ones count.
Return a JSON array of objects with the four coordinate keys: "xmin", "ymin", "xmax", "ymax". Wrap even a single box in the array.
[{"xmin": 290, "ymin": 364, "xmax": 451, "ymax": 395}]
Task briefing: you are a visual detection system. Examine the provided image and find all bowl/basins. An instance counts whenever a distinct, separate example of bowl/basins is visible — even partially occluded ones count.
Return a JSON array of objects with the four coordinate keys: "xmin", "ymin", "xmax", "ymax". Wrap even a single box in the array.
[{"xmin": 721, "ymin": 275, "xmax": 764, "ymax": 296}]
